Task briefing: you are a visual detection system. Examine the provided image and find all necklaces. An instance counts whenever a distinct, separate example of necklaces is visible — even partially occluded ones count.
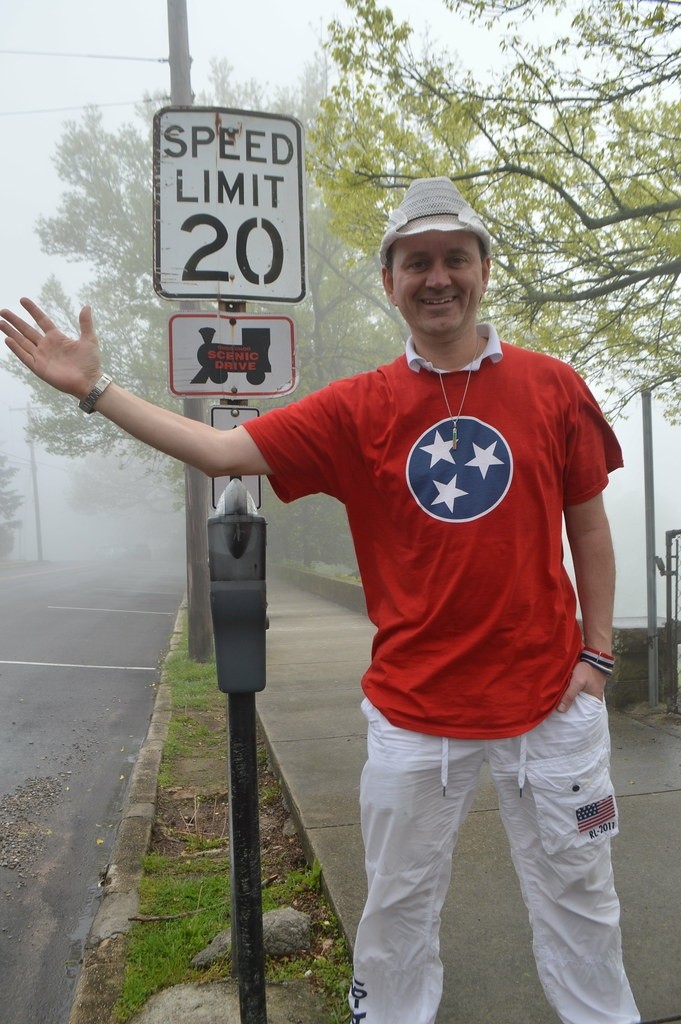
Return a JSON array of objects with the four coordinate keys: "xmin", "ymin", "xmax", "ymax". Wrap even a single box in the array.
[{"xmin": 436, "ymin": 343, "xmax": 480, "ymax": 450}]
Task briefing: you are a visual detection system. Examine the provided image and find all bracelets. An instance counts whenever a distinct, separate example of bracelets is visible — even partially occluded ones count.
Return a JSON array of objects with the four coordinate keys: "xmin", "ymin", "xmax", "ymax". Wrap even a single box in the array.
[
  {"xmin": 581, "ymin": 647, "xmax": 615, "ymax": 676},
  {"xmin": 78, "ymin": 374, "xmax": 113, "ymax": 414}
]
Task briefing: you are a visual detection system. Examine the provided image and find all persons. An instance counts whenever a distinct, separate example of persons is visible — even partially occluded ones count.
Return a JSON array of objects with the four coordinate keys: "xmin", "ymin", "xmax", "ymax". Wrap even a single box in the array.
[{"xmin": 0, "ymin": 175, "xmax": 640, "ymax": 1024}]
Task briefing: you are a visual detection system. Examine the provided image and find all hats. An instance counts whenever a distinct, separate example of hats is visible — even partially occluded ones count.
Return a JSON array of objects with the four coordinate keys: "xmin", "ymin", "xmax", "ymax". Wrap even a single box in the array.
[{"xmin": 380, "ymin": 177, "xmax": 491, "ymax": 267}]
